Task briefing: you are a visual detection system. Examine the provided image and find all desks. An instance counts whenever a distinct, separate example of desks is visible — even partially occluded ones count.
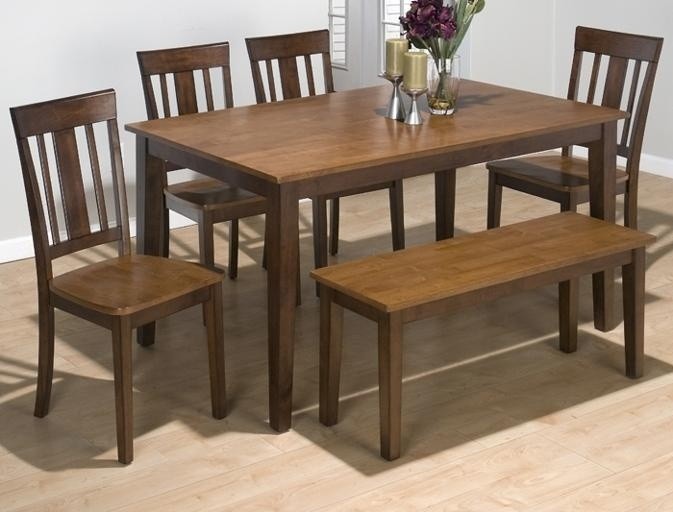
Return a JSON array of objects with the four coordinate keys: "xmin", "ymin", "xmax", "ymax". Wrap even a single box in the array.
[{"xmin": 125, "ymin": 77, "xmax": 630, "ymax": 434}]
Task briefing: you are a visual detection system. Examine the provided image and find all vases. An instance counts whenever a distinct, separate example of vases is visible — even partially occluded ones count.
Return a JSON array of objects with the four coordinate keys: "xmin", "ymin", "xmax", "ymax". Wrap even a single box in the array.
[{"xmin": 427, "ymin": 54, "xmax": 461, "ymax": 115}]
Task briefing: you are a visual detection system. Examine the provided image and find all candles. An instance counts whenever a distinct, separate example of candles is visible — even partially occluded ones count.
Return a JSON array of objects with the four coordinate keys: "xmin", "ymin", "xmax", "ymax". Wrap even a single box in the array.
[
  {"xmin": 386, "ymin": 39, "xmax": 409, "ymax": 75},
  {"xmin": 403, "ymin": 52, "xmax": 428, "ymax": 89}
]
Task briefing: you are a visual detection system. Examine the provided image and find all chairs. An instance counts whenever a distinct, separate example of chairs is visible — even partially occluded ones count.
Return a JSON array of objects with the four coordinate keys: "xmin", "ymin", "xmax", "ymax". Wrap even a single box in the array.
[
  {"xmin": 244, "ymin": 28, "xmax": 405, "ymax": 297},
  {"xmin": 9, "ymin": 88, "xmax": 225, "ymax": 465},
  {"xmin": 136, "ymin": 41, "xmax": 302, "ymax": 307},
  {"xmin": 486, "ymin": 26, "xmax": 663, "ymax": 230}
]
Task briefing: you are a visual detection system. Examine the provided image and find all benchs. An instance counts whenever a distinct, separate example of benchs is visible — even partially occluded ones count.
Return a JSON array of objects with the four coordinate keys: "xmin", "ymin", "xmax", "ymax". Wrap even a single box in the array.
[{"xmin": 310, "ymin": 211, "xmax": 657, "ymax": 461}]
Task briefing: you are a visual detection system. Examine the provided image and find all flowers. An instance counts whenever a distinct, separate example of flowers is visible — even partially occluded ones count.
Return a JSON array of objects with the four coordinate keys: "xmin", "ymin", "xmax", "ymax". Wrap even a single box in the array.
[{"xmin": 398, "ymin": 0, "xmax": 485, "ymax": 108}]
